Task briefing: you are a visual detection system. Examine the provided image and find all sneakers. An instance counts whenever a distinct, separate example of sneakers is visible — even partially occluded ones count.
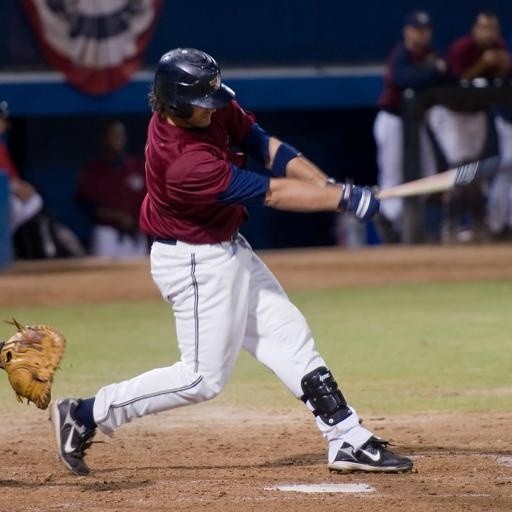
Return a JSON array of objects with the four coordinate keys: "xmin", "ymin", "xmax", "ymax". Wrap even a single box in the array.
[
  {"xmin": 50, "ymin": 396, "xmax": 92, "ymax": 479},
  {"xmin": 327, "ymin": 432, "xmax": 414, "ymax": 474}
]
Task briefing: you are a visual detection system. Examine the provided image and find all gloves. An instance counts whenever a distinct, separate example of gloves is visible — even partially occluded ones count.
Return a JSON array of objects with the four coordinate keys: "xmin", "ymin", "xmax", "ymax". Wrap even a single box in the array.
[{"xmin": 334, "ymin": 179, "xmax": 384, "ymax": 223}]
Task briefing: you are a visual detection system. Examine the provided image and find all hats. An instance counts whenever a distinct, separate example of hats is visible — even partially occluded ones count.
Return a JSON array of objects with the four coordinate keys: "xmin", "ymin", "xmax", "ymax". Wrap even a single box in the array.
[{"xmin": 406, "ymin": 10, "xmax": 435, "ymax": 29}]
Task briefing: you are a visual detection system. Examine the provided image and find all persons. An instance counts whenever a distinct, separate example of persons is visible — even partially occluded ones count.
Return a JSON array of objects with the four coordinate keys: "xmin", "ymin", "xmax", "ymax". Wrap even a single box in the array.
[
  {"xmin": 76, "ymin": 119, "xmax": 148, "ymax": 258},
  {"xmin": 484, "ymin": 103, "xmax": 512, "ymax": 233},
  {"xmin": 48, "ymin": 45, "xmax": 415, "ymax": 477},
  {"xmin": 0, "ymin": 99, "xmax": 44, "ymax": 231},
  {"xmin": 371, "ymin": 11, "xmax": 447, "ymax": 244},
  {"xmin": 424, "ymin": 10, "xmax": 509, "ymax": 243}
]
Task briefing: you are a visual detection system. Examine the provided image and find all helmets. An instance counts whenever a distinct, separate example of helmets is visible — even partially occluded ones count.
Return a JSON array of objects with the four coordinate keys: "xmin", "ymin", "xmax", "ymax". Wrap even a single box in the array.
[{"xmin": 153, "ymin": 46, "xmax": 236, "ymax": 111}]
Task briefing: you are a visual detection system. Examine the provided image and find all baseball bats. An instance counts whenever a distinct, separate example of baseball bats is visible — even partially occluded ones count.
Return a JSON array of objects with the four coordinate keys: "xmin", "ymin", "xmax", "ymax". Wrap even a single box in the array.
[{"xmin": 375, "ymin": 154, "xmax": 512, "ymax": 202}]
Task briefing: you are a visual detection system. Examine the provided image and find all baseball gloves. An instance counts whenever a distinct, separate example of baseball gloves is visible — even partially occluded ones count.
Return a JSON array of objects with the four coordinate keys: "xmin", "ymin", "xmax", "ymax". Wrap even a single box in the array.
[{"xmin": 0, "ymin": 318, "xmax": 66, "ymax": 408}]
